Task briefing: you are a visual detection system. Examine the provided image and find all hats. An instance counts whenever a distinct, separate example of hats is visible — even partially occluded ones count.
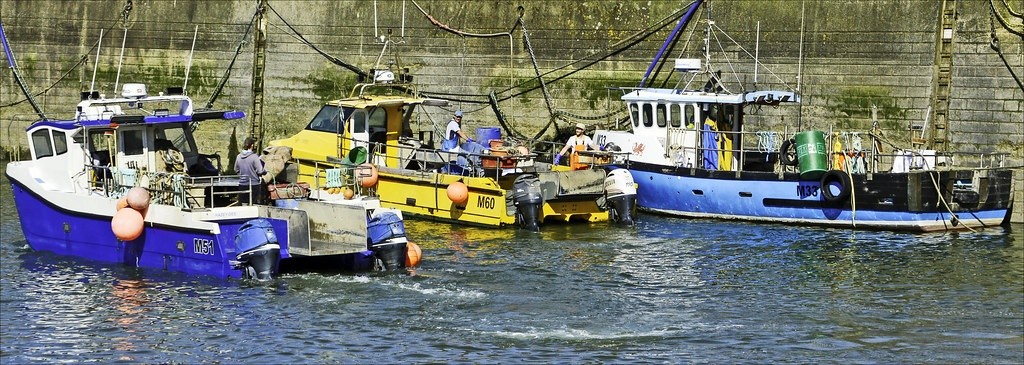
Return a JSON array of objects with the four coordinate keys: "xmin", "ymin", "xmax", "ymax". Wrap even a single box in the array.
[
  {"xmin": 576, "ymin": 123, "xmax": 585, "ymax": 130},
  {"xmin": 454, "ymin": 111, "xmax": 463, "ymax": 118}
]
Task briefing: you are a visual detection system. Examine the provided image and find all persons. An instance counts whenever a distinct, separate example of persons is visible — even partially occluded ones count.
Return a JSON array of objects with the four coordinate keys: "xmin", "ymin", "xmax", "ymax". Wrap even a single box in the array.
[
  {"xmin": 234, "ymin": 137, "xmax": 267, "ymax": 206},
  {"xmin": 442, "ymin": 111, "xmax": 473, "ymax": 175},
  {"xmin": 554, "ymin": 122, "xmax": 604, "ymax": 171}
]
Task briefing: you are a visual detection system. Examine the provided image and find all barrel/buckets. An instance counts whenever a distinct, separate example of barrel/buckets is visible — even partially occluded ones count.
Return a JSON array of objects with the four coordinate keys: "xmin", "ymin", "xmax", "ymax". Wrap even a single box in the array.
[
  {"xmin": 796, "ymin": 131, "xmax": 828, "ymax": 178},
  {"xmin": 341, "ymin": 146, "xmax": 367, "ymax": 169}
]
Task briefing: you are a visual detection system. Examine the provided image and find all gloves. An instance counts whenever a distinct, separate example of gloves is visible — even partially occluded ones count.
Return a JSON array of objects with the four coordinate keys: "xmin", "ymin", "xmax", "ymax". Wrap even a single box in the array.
[
  {"xmin": 599, "ymin": 146, "xmax": 605, "ymax": 151},
  {"xmin": 466, "ymin": 138, "xmax": 474, "ymax": 142},
  {"xmin": 554, "ymin": 153, "xmax": 561, "ymax": 165}
]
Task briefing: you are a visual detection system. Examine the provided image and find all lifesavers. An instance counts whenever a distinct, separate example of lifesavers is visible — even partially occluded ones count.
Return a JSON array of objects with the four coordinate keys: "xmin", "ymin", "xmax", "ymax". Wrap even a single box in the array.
[
  {"xmin": 821, "ymin": 169, "xmax": 852, "ymax": 203},
  {"xmin": 781, "ymin": 139, "xmax": 798, "ymax": 165}
]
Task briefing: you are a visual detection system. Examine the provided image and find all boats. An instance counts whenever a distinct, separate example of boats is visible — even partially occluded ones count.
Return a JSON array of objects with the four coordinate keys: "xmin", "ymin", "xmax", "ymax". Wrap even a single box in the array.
[
  {"xmin": 2, "ymin": 25, "xmax": 409, "ymax": 282},
  {"xmin": 263, "ymin": 0, "xmax": 641, "ymax": 234},
  {"xmin": 567, "ymin": 0, "xmax": 1016, "ymax": 233}
]
